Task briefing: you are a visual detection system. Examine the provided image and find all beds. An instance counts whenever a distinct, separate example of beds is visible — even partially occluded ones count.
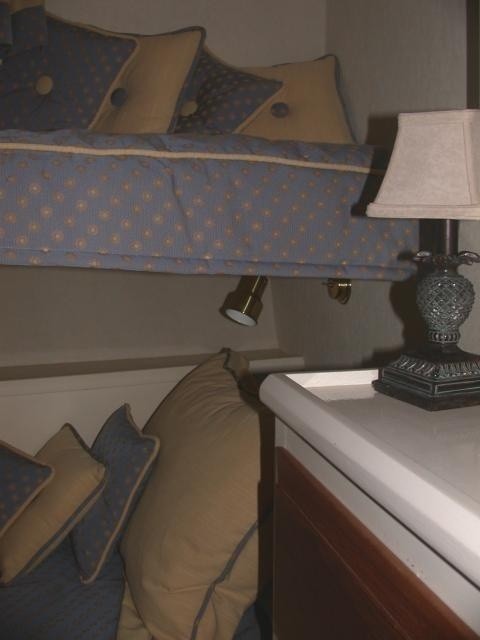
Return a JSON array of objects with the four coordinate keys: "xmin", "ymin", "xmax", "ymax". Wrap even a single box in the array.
[{"xmin": 0, "ymin": 121, "xmax": 425, "ymax": 639}]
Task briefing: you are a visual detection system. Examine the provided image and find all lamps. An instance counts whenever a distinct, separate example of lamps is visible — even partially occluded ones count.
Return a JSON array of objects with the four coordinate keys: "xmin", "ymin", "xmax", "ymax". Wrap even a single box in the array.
[
  {"xmin": 362, "ymin": 111, "xmax": 480, "ymax": 410},
  {"xmin": 220, "ymin": 276, "xmax": 351, "ymax": 327}
]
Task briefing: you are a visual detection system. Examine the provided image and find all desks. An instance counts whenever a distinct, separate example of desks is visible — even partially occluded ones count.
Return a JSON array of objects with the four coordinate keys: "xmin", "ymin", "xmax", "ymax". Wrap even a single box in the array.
[{"xmin": 257, "ymin": 367, "xmax": 477, "ymax": 636}]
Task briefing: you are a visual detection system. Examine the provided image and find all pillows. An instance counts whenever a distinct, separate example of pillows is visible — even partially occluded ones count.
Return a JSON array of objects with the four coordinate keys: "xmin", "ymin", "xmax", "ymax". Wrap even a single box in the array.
[
  {"xmin": 75, "ymin": 397, "xmax": 165, "ymax": 585},
  {"xmin": 172, "ymin": 45, "xmax": 284, "ymax": 142},
  {"xmin": 226, "ymin": 53, "xmax": 356, "ymax": 145},
  {"xmin": 2, "ymin": 435, "xmax": 59, "ymax": 533},
  {"xmin": 71, "ymin": 20, "xmax": 210, "ymax": 139},
  {"xmin": 4, "ymin": 2, "xmax": 139, "ymax": 137},
  {"xmin": 110, "ymin": 348, "xmax": 265, "ymax": 639},
  {"xmin": 3, "ymin": 423, "xmax": 110, "ymax": 587}
]
